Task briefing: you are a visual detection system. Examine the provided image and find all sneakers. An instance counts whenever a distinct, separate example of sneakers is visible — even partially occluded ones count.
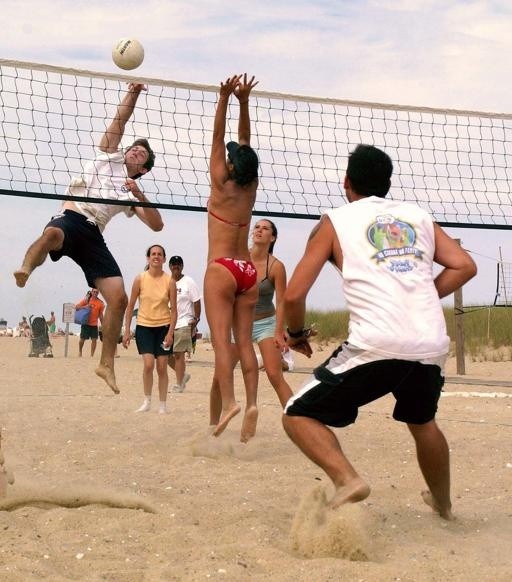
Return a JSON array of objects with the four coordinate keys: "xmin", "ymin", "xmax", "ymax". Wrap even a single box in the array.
[{"xmin": 172, "ymin": 373, "xmax": 189, "ymax": 393}]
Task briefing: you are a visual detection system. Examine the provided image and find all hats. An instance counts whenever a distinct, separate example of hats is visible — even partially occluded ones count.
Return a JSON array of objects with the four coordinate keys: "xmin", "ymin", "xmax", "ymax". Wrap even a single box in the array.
[
  {"xmin": 169, "ymin": 256, "xmax": 183, "ymax": 265},
  {"xmin": 226, "ymin": 141, "xmax": 258, "ymax": 173}
]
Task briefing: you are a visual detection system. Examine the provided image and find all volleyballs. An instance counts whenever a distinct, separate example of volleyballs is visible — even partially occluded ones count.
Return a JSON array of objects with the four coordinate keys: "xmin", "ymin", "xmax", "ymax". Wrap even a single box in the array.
[{"xmin": 112, "ymin": 38, "xmax": 144, "ymax": 70}]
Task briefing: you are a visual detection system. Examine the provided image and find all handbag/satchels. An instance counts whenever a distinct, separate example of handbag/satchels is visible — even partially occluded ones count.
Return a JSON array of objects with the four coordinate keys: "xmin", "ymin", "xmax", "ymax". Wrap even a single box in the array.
[{"xmin": 75, "ymin": 309, "xmax": 91, "ymax": 325}]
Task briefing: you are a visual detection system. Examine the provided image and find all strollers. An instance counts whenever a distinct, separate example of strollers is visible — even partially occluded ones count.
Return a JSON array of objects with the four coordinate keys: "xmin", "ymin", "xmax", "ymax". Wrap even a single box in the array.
[{"xmin": 26, "ymin": 313, "xmax": 54, "ymax": 358}]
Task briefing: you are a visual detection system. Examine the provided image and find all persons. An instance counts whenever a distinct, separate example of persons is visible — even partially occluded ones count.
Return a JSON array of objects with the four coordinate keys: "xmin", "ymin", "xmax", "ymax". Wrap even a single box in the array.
[
  {"xmin": 167, "ymin": 255, "xmax": 202, "ymax": 394},
  {"xmin": 202, "ymin": 71, "xmax": 260, "ymax": 443},
  {"xmin": 0, "ymin": 311, "xmax": 57, "ymax": 337},
  {"xmin": 208, "ymin": 218, "xmax": 295, "ymax": 425},
  {"xmin": 113, "ymin": 344, "xmax": 120, "ymax": 359},
  {"xmin": 259, "ymin": 330, "xmax": 296, "ymax": 374},
  {"xmin": 74, "ymin": 287, "xmax": 106, "ymax": 357},
  {"xmin": 121, "ymin": 244, "xmax": 178, "ymax": 416},
  {"xmin": 13, "ymin": 80, "xmax": 164, "ymax": 396},
  {"xmin": 280, "ymin": 143, "xmax": 480, "ymax": 521},
  {"xmin": 191, "ymin": 326, "xmax": 200, "ymax": 354}
]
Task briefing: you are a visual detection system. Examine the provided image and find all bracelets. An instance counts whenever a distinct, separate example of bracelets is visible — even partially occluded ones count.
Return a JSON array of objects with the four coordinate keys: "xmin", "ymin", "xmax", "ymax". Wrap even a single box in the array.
[
  {"xmin": 286, "ymin": 325, "xmax": 305, "ymax": 338},
  {"xmin": 192, "ymin": 316, "xmax": 201, "ymax": 321}
]
